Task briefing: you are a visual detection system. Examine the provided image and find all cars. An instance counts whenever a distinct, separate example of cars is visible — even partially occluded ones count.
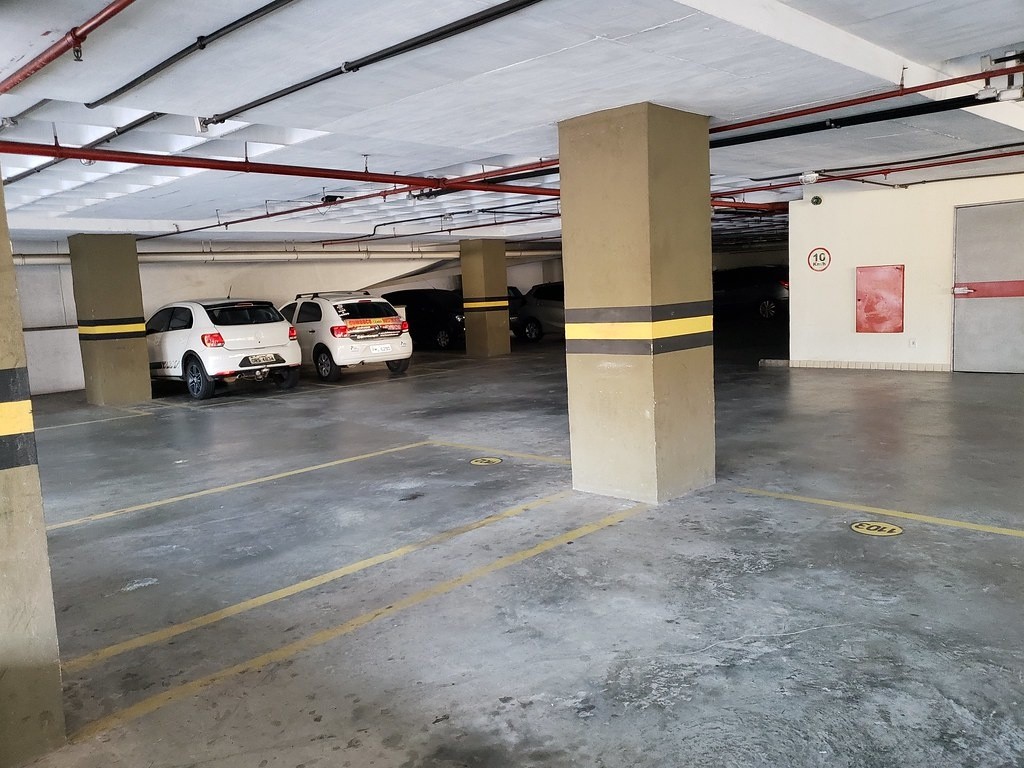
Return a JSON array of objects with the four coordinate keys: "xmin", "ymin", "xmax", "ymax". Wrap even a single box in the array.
[
  {"xmin": 277, "ymin": 290, "xmax": 413, "ymax": 382},
  {"xmin": 452, "ymin": 285, "xmax": 523, "ymax": 337}
]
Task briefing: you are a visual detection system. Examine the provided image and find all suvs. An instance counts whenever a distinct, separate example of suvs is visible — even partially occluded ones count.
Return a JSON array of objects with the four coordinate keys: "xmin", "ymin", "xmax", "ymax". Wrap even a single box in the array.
[
  {"xmin": 145, "ymin": 297, "xmax": 303, "ymax": 400},
  {"xmin": 713, "ymin": 264, "xmax": 789, "ymax": 320},
  {"xmin": 520, "ymin": 281, "xmax": 566, "ymax": 345}
]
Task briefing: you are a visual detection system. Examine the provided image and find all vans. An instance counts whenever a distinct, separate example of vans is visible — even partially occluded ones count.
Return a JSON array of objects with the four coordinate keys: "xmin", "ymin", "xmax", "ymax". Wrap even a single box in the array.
[{"xmin": 381, "ymin": 288, "xmax": 521, "ymax": 354}]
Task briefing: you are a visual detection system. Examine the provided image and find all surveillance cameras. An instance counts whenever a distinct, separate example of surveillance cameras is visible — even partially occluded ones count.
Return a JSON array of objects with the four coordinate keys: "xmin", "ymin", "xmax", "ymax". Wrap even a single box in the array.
[
  {"xmin": 996, "ymin": 87, "xmax": 1024, "ymax": 102},
  {"xmin": 977, "ymin": 88, "xmax": 997, "ymax": 100}
]
